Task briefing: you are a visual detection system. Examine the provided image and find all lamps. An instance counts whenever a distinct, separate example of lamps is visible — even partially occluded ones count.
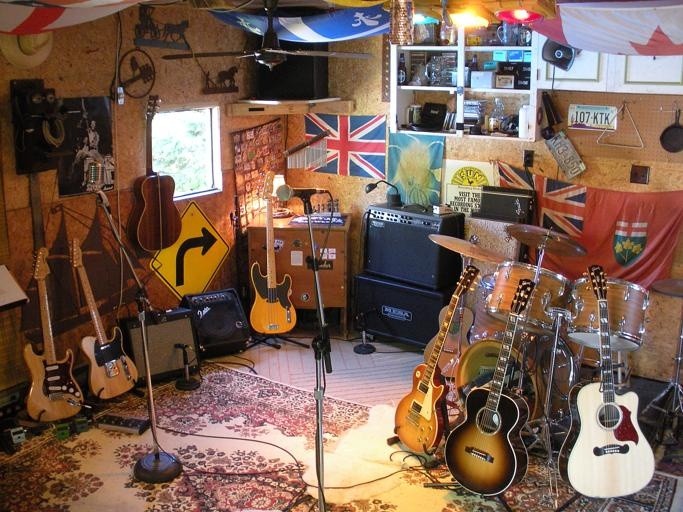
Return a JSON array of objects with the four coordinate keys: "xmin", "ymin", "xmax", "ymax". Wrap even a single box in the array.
[
  {"xmin": 265, "ymin": 173, "xmax": 291, "ymax": 218},
  {"xmin": 494, "ymin": 1, "xmax": 541, "ymax": 28},
  {"xmin": 363, "ymin": 180, "xmax": 402, "ymax": 210}
]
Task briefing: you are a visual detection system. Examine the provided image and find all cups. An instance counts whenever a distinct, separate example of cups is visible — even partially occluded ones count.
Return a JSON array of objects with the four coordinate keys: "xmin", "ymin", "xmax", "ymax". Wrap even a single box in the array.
[
  {"xmin": 496, "ymin": 19, "xmax": 518, "ymax": 45},
  {"xmin": 466, "ymin": 34, "xmax": 481, "ymax": 46},
  {"xmin": 469, "ymin": 126, "xmax": 481, "ymax": 135},
  {"xmin": 406, "ymin": 105, "xmax": 422, "ymax": 127},
  {"xmin": 423, "ymin": 55, "xmax": 447, "ymax": 87}
]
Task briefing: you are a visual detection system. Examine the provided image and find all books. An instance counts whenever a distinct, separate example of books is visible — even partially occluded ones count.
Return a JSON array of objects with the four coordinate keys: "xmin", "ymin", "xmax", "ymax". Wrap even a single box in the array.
[{"xmin": 445, "ymin": 97, "xmax": 486, "ymax": 134}]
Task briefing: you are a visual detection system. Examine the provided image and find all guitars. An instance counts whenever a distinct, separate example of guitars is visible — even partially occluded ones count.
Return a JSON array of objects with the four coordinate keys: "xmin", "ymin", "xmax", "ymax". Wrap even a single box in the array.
[
  {"xmin": 69, "ymin": 236, "xmax": 138, "ymax": 399},
  {"xmin": 558, "ymin": 263, "xmax": 655, "ymax": 498},
  {"xmin": 444, "ymin": 279, "xmax": 535, "ymax": 497},
  {"xmin": 126, "ymin": 94, "xmax": 181, "ymax": 251},
  {"xmin": 395, "ymin": 265, "xmax": 480, "ymax": 456},
  {"xmin": 23, "ymin": 247, "xmax": 84, "ymax": 423},
  {"xmin": 424, "ymin": 234, "xmax": 479, "ymax": 379},
  {"xmin": 250, "ymin": 170, "xmax": 297, "ymax": 334}
]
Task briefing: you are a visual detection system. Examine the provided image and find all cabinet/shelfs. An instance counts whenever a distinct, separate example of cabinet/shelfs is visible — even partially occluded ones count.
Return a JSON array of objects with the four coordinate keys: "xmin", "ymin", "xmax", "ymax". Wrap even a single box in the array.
[
  {"xmin": 246, "ymin": 211, "xmax": 354, "ymax": 339},
  {"xmin": 390, "ymin": 45, "xmax": 542, "ymax": 142},
  {"xmin": 533, "ymin": 2, "xmax": 683, "ymax": 96}
]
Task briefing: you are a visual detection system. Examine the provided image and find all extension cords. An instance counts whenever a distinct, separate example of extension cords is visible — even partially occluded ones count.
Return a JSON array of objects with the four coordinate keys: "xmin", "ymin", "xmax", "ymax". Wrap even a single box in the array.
[{"xmin": 97, "ymin": 414, "xmax": 149, "ymax": 435}]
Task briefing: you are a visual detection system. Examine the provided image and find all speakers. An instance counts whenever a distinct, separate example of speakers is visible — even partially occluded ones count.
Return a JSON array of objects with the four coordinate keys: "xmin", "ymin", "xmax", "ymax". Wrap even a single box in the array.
[
  {"xmin": 178, "ymin": 288, "xmax": 250, "ymax": 360},
  {"xmin": 353, "ymin": 274, "xmax": 459, "ymax": 351},
  {"xmin": 118, "ymin": 307, "xmax": 198, "ymax": 388},
  {"xmin": 363, "ymin": 202, "xmax": 465, "ymax": 293},
  {"xmin": 247, "ymin": 6, "xmax": 330, "ymax": 100}
]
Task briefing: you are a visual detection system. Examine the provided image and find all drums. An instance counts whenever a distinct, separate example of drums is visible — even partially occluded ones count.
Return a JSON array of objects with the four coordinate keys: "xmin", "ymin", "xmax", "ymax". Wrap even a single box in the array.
[
  {"xmin": 567, "ymin": 277, "xmax": 650, "ymax": 352},
  {"xmin": 477, "ymin": 260, "xmax": 575, "ymax": 339},
  {"xmin": 454, "ymin": 325, "xmax": 580, "ymax": 433}
]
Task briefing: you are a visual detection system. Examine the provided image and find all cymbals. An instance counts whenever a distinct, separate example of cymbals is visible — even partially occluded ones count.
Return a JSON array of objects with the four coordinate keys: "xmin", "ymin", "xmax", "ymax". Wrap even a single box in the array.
[
  {"xmin": 428, "ymin": 233, "xmax": 509, "ymax": 265},
  {"xmin": 505, "ymin": 223, "xmax": 587, "ymax": 257}
]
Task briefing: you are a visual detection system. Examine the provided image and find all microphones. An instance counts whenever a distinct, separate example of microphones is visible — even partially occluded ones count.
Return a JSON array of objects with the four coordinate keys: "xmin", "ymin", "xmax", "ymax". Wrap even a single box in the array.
[
  {"xmin": 86, "ymin": 162, "xmax": 105, "ymax": 193},
  {"xmin": 174, "ymin": 343, "xmax": 190, "ymax": 348},
  {"xmin": 276, "ymin": 184, "xmax": 329, "ymax": 202}
]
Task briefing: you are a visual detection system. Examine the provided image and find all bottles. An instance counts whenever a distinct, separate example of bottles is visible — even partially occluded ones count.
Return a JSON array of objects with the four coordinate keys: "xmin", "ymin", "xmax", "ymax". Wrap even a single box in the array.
[
  {"xmin": 487, "ymin": 109, "xmax": 498, "ymax": 132},
  {"xmin": 464, "ymin": 62, "xmax": 470, "ymax": 87},
  {"xmin": 440, "ymin": 20, "xmax": 449, "ymax": 47},
  {"xmin": 471, "ymin": 54, "xmax": 479, "ymax": 71},
  {"xmin": 519, "ymin": 23, "xmax": 530, "ymax": 46},
  {"xmin": 451, "ymin": 67, "xmax": 456, "ymax": 86},
  {"xmin": 397, "ymin": 52, "xmax": 407, "ymax": 85}
]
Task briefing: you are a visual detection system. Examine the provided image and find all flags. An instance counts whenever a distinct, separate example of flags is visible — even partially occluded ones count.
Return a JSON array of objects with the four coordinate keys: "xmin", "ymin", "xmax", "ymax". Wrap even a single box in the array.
[
  {"xmin": 385, "ymin": 127, "xmax": 445, "ymax": 207},
  {"xmin": 488, "ymin": 158, "xmax": 682, "ymax": 292},
  {"xmin": 304, "ymin": 112, "xmax": 386, "ymax": 180},
  {"xmin": 0, "ymin": 0, "xmax": 146, "ymax": 35},
  {"xmin": 527, "ymin": 2, "xmax": 682, "ymax": 55},
  {"xmin": 207, "ymin": 3, "xmax": 439, "ymax": 44}
]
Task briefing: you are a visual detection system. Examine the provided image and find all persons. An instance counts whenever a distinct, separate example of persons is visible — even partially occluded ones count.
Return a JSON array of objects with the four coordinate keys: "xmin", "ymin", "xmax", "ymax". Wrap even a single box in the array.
[{"xmin": 71, "ymin": 111, "xmax": 104, "ymax": 161}]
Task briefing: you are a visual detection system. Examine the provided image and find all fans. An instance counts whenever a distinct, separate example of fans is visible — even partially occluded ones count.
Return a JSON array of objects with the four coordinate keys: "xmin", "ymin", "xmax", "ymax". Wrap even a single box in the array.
[{"xmin": 160, "ymin": 0, "xmax": 375, "ymax": 71}]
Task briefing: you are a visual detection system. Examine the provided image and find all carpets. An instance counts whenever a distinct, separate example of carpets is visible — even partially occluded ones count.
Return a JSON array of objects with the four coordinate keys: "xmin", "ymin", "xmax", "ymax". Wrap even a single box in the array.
[{"xmin": 0, "ymin": 360, "xmax": 683, "ymax": 512}]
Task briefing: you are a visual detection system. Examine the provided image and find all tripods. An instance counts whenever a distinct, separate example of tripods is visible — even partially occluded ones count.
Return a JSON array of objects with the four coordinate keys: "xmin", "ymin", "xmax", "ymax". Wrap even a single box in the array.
[
  {"xmin": 521, "ymin": 313, "xmax": 569, "ymax": 466},
  {"xmin": 640, "ymin": 323, "xmax": 683, "ymax": 435},
  {"xmin": 439, "ymin": 258, "xmax": 474, "ymax": 415}
]
